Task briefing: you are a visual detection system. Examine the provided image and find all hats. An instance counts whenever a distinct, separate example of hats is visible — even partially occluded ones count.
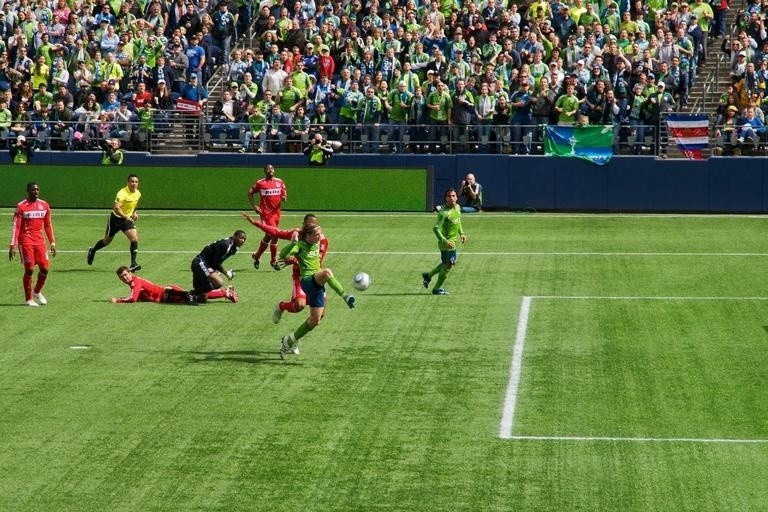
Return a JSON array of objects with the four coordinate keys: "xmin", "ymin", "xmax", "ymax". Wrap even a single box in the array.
[
  {"xmin": 728, "ymin": 105, "xmax": 737, "ymax": 111},
  {"xmin": 738, "ymin": 51, "xmax": 747, "ymax": 56},
  {"xmin": 0, "ymin": 4, "xmax": 198, "ymax": 84},
  {"xmin": 230, "ymin": 1, "xmax": 698, "ymax": 87}
]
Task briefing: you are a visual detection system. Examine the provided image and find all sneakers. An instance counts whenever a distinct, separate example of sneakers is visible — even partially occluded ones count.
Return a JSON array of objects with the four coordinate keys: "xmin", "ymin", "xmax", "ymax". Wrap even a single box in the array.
[
  {"xmin": 433, "ymin": 288, "xmax": 449, "ymax": 295},
  {"xmin": 25, "ymin": 299, "xmax": 38, "ymax": 306},
  {"xmin": 188, "ymin": 253, "xmax": 355, "ymax": 360},
  {"xmin": 88, "ymin": 247, "xmax": 94, "ymax": 264},
  {"xmin": 32, "ymin": 289, "xmax": 47, "ymax": 304},
  {"xmin": 128, "ymin": 264, "xmax": 141, "ymax": 272},
  {"xmin": 423, "ymin": 273, "xmax": 431, "ymax": 289}
]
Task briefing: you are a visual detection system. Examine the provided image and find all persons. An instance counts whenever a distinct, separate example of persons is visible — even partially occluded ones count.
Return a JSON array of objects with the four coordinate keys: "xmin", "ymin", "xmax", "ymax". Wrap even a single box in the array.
[
  {"xmin": 177, "ymin": 73, "xmax": 208, "ymax": 150},
  {"xmin": 110, "ymin": 266, "xmax": 238, "ymax": 306},
  {"xmin": 305, "ymin": 134, "xmax": 333, "ymax": 166},
  {"xmin": 8, "ymin": 181, "xmax": 57, "ymax": 306},
  {"xmin": 99, "ymin": 139, "xmax": 123, "ymax": 165},
  {"xmin": 1, "ymin": 1, "xmax": 259, "ymax": 150},
  {"xmin": 713, "ymin": 1, "xmax": 764, "ymax": 156},
  {"xmin": 241, "ymin": 210, "xmax": 329, "ymax": 325},
  {"xmin": 247, "ymin": 164, "xmax": 287, "ymax": 271},
  {"xmin": 457, "ymin": 171, "xmax": 483, "ymax": 214},
  {"xmin": 277, "ymin": 224, "xmax": 356, "ymax": 360},
  {"xmin": 206, "ymin": 1, "xmax": 728, "ymax": 153},
  {"xmin": 9, "ymin": 135, "xmax": 34, "ymax": 165},
  {"xmin": 87, "ymin": 174, "xmax": 142, "ymax": 271},
  {"xmin": 420, "ymin": 188, "xmax": 466, "ymax": 295},
  {"xmin": 189, "ymin": 230, "xmax": 248, "ymax": 303}
]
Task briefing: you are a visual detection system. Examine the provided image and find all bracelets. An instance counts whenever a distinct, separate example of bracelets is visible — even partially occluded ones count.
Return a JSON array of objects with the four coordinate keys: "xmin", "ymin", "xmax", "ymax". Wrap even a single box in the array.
[{"xmin": 50, "ymin": 242, "xmax": 55, "ymax": 247}]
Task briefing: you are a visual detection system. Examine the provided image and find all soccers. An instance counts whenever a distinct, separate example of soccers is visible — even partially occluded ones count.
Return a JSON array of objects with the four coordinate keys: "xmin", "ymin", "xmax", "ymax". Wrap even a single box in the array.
[{"xmin": 353, "ymin": 273, "xmax": 370, "ymax": 290}]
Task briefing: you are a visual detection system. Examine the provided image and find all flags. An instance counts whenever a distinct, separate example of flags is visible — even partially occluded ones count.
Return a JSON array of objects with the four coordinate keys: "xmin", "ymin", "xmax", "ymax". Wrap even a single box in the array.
[
  {"xmin": 544, "ymin": 125, "xmax": 614, "ymax": 165},
  {"xmin": 664, "ymin": 115, "xmax": 709, "ymax": 161}
]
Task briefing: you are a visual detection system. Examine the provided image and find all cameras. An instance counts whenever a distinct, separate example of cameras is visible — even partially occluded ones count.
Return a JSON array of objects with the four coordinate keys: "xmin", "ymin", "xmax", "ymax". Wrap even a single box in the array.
[
  {"xmin": 465, "ymin": 180, "xmax": 469, "ymax": 186},
  {"xmin": 20, "ymin": 140, "xmax": 34, "ymax": 147},
  {"xmin": 314, "ymin": 139, "xmax": 321, "ymax": 144}
]
